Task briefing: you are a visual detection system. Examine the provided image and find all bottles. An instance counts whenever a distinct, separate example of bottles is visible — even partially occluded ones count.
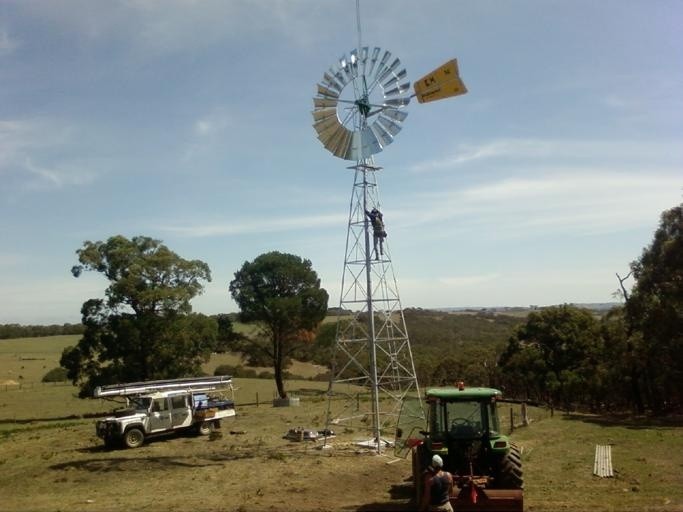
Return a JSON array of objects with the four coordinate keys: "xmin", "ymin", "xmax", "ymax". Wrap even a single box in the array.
[{"xmin": 432, "ymin": 454, "xmax": 443, "ymax": 468}]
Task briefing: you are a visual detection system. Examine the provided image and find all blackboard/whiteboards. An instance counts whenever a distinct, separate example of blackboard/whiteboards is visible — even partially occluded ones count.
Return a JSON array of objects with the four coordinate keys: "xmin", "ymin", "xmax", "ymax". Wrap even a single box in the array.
[
  {"xmin": 389, "ymin": 379, "xmax": 525, "ymax": 512},
  {"xmin": 85, "ymin": 374, "xmax": 241, "ymax": 451}
]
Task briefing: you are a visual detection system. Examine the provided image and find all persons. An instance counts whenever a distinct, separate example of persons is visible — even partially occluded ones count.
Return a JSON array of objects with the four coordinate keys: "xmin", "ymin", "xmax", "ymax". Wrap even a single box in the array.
[
  {"xmin": 196, "ymin": 401, "xmax": 203, "ymax": 409},
  {"xmin": 366, "ymin": 209, "xmax": 387, "ymax": 261},
  {"xmin": 420, "ymin": 453, "xmax": 455, "ymax": 512}
]
